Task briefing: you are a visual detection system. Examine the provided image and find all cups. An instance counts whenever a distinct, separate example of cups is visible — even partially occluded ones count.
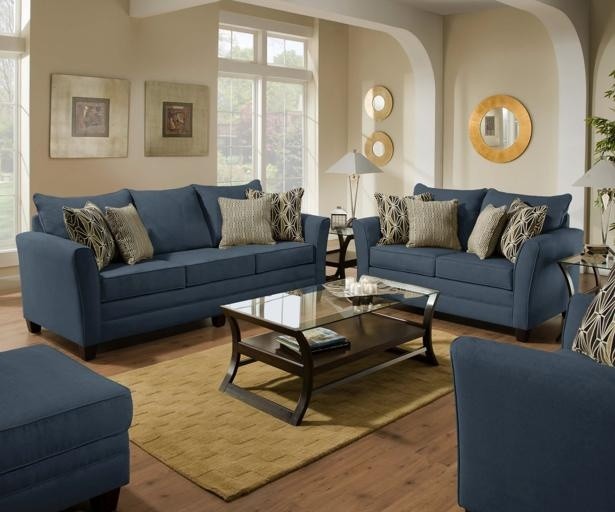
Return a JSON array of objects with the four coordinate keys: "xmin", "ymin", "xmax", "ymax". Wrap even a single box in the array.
[{"xmin": 345, "ymin": 275, "xmax": 377, "ymax": 295}]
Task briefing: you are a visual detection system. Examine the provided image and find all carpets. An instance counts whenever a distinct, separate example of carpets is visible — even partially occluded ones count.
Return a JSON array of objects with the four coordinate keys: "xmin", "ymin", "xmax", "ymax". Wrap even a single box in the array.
[{"xmin": 106, "ymin": 328, "xmax": 460, "ymax": 503}]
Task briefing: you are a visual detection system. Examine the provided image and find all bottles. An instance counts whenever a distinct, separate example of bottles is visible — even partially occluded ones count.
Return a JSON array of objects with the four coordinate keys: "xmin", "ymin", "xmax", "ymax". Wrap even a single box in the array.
[{"xmin": 332, "ymin": 206, "xmax": 347, "ymax": 226}]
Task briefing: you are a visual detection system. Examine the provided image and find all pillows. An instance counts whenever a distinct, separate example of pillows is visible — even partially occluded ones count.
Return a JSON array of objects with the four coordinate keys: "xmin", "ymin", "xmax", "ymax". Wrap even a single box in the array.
[
  {"xmin": 375, "ymin": 182, "xmax": 573, "ymax": 262},
  {"xmin": 32, "ymin": 178, "xmax": 306, "ymax": 272},
  {"xmin": 571, "ymin": 258, "xmax": 615, "ymax": 366}
]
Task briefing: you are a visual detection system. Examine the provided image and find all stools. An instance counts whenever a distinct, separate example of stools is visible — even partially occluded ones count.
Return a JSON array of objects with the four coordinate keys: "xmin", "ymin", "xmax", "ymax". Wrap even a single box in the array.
[{"xmin": 0, "ymin": 343, "xmax": 133, "ymax": 512}]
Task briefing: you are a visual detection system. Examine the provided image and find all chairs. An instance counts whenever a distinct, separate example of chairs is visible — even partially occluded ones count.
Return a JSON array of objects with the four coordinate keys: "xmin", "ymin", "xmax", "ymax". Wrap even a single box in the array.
[{"xmin": 450, "ymin": 292, "xmax": 615, "ymax": 511}]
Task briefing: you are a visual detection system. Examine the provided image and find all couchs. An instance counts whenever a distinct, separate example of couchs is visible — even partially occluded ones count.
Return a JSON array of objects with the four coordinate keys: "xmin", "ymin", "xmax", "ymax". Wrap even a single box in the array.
[
  {"xmin": 15, "ymin": 214, "xmax": 330, "ymax": 360},
  {"xmin": 351, "ymin": 210, "xmax": 584, "ymax": 342}
]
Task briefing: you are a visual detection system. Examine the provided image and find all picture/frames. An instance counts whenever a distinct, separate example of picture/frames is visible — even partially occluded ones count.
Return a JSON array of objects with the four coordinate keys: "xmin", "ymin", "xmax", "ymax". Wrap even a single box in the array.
[
  {"xmin": 48, "ymin": 72, "xmax": 131, "ymax": 158},
  {"xmin": 144, "ymin": 79, "xmax": 211, "ymax": 156}
]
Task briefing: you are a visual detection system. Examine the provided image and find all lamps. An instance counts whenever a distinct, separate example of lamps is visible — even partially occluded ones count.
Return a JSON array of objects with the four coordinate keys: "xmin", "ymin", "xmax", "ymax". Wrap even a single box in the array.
[
  {"xmin": 324, "ymin": 149, "xmax": 384, "ymax": 228},
  {"xmin": 571, "ymin": 158, "xmax": 615, "ymax": 253}
]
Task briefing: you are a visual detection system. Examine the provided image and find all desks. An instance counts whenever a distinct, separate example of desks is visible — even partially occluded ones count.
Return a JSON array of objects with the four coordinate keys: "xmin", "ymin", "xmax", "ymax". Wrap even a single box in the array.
[
  {"xmin": 556, "ymin": 251, "xmax": 615, "ymax": 342},
  {"xmin": 324, "ymin": 227, "xmax": 358, "ymax": 281}
]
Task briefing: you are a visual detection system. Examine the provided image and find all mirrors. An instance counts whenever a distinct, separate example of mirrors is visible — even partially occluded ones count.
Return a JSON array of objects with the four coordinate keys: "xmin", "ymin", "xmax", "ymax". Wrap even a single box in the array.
[
  {"xmin": 364, "ymin": 84, "xmax": 393, "ymax": 121},
  {"xmin": 364, "ymin": 130, "xmax": 393, "ymax": 167},
  {"xmin": 467, "ymin": 93, "xmax": 533, "ymax": 163}
]
